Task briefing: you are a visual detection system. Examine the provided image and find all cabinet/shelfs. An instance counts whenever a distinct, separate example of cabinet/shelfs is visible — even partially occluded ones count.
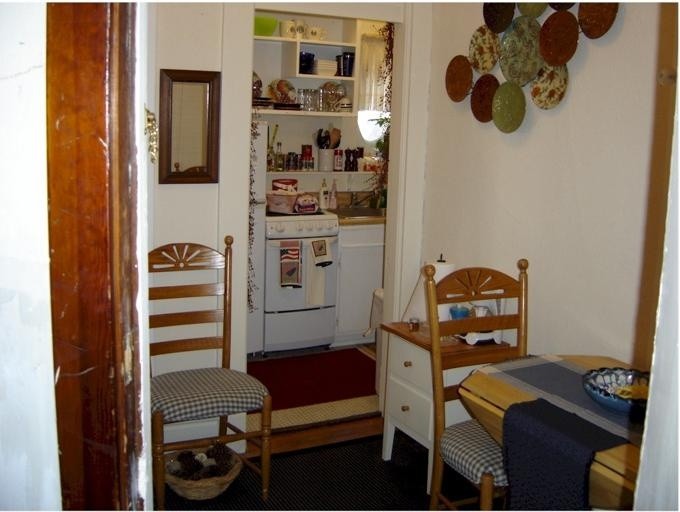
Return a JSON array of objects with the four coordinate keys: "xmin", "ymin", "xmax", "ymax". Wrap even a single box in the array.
[
  {"xmin": 252, "ymin": 5, "xmax": 394, "ymax": 118},
  {"xmin": 329, "ymin": 214, "xmax": 388, "ymax": 347},
  {"xmin": 375, "ymin": 319, "xmax": 518, "ymax": 497}
]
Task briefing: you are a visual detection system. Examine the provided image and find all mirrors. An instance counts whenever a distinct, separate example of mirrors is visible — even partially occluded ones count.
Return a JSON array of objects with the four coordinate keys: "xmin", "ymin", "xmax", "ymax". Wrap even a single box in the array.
[{"xmin": 156, "ymin": 64, "xmax": 223, "ymax": 186}]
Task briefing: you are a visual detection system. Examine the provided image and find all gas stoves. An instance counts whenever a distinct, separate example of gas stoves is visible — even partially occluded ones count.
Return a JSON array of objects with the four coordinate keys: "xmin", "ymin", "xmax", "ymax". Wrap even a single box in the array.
[{"xmin": 260, "ymin": 200, "xmax": 340, "ymax": 240}]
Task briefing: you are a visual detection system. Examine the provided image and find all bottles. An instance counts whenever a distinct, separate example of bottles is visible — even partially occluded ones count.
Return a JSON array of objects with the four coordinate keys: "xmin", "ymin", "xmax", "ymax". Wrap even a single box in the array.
[
  {"xmin": 409, "ymin": 319, "xmax": 420, "ymax": 332},
  {"xmin": 334, "ymin": 149, "xmax": 342, "ymax": 171},
  {"xmin": 274, "ymin": 141, "xmax": 285, "ymax": 171},
  {"xmin": 319, "ymin": 178, "xmax": 338, "ymax": 210},
  {"xmin": 287, "ymin": 150, "xmax": 315, "ymax": 171},
  {"xmin": 344, "ymin": 147, "xmax": 358, "ymax": 172}
]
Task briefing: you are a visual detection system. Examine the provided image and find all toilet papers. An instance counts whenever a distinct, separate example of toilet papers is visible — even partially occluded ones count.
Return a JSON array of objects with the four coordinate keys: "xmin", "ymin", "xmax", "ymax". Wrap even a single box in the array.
[{"xmin": 401, "ymin": 262, "xmax": 457, "ymax": 323}]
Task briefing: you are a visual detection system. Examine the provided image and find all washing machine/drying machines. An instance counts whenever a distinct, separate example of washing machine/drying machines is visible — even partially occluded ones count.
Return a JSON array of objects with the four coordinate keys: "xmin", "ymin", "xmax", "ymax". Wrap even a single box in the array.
[{"xmin": 265, "ymin": 209, "xmax": 339, "ymax": 356}]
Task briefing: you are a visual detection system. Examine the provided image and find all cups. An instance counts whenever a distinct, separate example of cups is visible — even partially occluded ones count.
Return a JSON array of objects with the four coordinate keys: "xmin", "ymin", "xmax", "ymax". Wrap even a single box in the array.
[
  {"xmin": 278, "ymin": 18, "xmax": 327, "ymax": 41},
  {"xmin": 299, "ymin": 51, "xmax": 315, "ymax": 73},
  {"xmin": 317, "ymin": 147, "xmax": 335, "ymax": 171},
  {"xmin": 333, "ymin": 96, "xmax": 352, "ymax": 112},
  {"xmin": 358, "ymin": 156, "xmax": 384, "ymax": 171},
  {"xmin": 337, "ymin": 54, "xmax": 343, "ymax": 75},
  {"xmin": 297, "ymin": 87, "xmax": 328, "ymax": 111},
  {"xmin": 342, "ymin": 51, "xmax": 355, "ymax": 77}
]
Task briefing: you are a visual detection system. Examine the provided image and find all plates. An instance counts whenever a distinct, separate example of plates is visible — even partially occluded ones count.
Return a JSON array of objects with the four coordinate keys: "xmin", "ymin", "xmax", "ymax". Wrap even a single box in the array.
[
  {"xmin": 252, "ymin": 72, "xmax": 263, "ymax": 97},
  {"xmin": 270, "ymin": 80, "xmax": 297, "ymax": 103},
  {"xmin": 273, "ymin": 102, "xmax": 299, "ymax": 110},
  {"xmin": 312, "ymin": 59, "xmax": 337, "ymax": 76}
]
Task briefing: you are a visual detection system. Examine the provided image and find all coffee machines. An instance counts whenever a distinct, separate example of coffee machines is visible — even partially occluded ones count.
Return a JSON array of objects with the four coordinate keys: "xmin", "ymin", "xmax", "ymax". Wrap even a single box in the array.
[{"xmin": 452, "ymin": 288, "xmax": 507, "ymax": 346}]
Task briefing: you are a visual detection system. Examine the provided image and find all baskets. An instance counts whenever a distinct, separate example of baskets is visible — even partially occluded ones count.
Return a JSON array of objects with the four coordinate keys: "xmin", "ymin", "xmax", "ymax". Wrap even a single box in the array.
[{"xmin": 163, "ymin": 448, "xmax": 243, "ymax": 500}]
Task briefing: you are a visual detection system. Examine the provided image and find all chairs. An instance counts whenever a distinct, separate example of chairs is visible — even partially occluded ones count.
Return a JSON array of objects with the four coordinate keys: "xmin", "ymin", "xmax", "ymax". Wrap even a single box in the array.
[
  {"xmin": 142, "ymin": 231, "xmax": 279, "ymax": 511},
  {"xmin": 419, "ymin": 255, "xmax": 544, "ymax": 511}
]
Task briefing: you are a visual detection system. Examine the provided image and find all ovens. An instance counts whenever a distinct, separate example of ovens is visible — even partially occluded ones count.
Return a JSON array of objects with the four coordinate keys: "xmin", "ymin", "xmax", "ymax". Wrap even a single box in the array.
[{"xmin": 264, "ymin": 237, "xmax": 338, "ymax": 351}]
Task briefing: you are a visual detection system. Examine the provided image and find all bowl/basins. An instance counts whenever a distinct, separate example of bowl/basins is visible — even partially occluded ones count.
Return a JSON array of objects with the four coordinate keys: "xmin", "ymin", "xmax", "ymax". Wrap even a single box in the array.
[
  {"xmin": 255, "ymin": 14, "xmax": 276, "ymax": 37},
  {"xmin": 252, "ymin": 96, "xmax": 273, "ymax": 109},
  {"xmin": 581, "ymin": 366, "xmax": 652, "ymax": 417}
]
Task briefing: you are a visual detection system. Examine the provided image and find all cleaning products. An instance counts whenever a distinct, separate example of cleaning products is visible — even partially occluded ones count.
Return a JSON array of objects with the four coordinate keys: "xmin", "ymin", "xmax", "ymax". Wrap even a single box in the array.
[
  {"xmin": 319, "ymin": 178, "xmax": 329, "ymax": 209},
  {"xmin": 329, "ymin": 179, "xmax": 338, "ymax": 210}
]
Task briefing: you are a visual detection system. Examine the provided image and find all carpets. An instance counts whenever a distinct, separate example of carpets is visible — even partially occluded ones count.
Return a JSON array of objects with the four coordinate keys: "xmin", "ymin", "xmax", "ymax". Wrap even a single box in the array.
[
  {"xmin": 241, "ymin": 343, "xmax": 378, "ymax": 416},
  {"xmin": 154, "ymin": 424, "xmax": 509, "ymax": 512}
]
